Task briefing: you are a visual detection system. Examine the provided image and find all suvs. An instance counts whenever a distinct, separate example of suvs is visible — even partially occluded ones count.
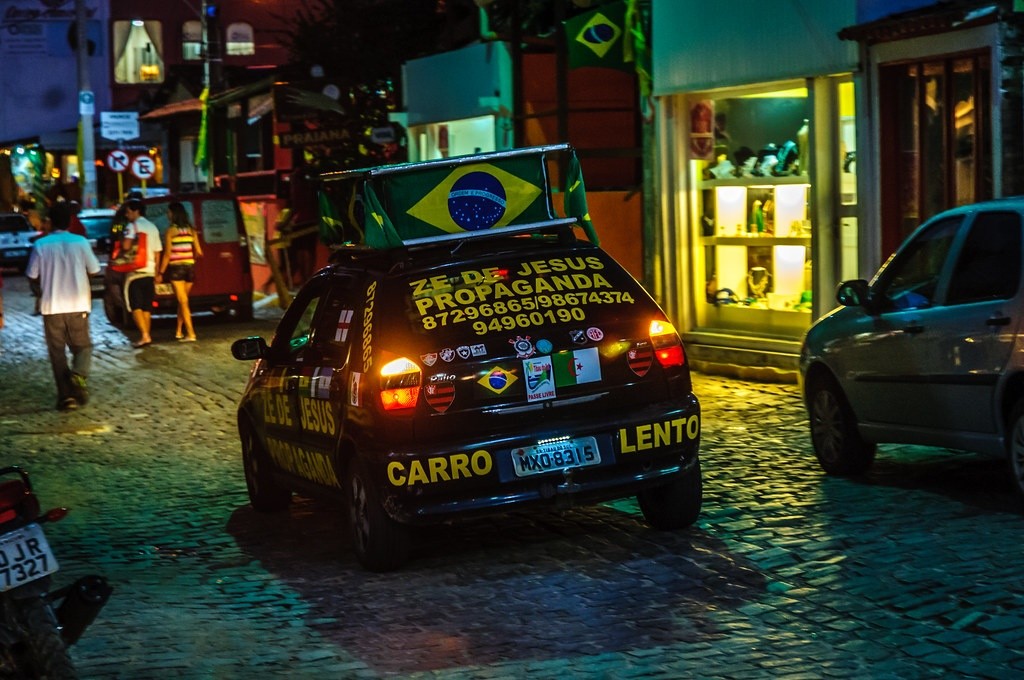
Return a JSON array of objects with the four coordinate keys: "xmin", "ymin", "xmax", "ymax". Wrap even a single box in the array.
[{"xmin": 231, "ymin": 222, "xmax": 705, "ymax": 568}]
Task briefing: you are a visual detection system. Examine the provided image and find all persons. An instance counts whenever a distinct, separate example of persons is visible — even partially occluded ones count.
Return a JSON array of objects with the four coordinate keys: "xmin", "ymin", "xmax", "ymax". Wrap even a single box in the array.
[
  {"xmin": 121, "ymin": 198, "xmax": 163, "ymax": 347},
  {"xmin": 156, "ymin": 202, "xmax": 203, "ymax": 342},
  {"xmin": 26, "ymin": 203, "xmax": 102, "ymax": 411}
]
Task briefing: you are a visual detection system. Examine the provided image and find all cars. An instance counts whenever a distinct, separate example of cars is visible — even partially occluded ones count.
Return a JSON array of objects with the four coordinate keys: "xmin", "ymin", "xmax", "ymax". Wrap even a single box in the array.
[
  {"xmin": 78, "ymin": 208, "xmax": 118, "ymax": 278},
  {"xmin": 795, "ymin": 198, "xmax": 1024, "ymax": 506},
  {"xmin": 0, "ymin": 212, "xmax": 48, "ymax": 267}
]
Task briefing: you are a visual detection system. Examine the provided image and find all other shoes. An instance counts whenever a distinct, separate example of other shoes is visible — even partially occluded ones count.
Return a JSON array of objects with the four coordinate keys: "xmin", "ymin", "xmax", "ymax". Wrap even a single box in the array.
[
  {"xmin": 70, "ymin": 374, "xmax": 89, "ymax": 405},
  {"xmin": 55, "ymin": 396, "xmax": 77, "ymax": 412}
]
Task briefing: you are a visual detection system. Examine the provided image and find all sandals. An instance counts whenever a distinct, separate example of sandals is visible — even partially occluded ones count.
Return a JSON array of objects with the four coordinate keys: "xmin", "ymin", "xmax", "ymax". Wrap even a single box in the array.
[
  {"xmin": 179, "ymin": 338, "xmax": 197, "ymax": 343},
  {"xmin": 175, "ymin": 334, "xmax": 184, "ymax": 339}
]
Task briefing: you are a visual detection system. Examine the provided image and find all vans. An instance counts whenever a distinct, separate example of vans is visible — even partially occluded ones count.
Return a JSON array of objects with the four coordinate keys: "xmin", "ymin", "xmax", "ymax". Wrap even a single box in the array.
[{"xmin": 100, "ymin": 189, "xmax": 256, "ymax": 326}]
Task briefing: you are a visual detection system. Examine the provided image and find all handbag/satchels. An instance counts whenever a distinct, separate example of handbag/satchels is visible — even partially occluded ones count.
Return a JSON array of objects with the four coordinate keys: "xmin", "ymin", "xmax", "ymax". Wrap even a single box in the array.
[{"xmin": 108, "ymin": 221, "xmax": 149, "ymax": 272}]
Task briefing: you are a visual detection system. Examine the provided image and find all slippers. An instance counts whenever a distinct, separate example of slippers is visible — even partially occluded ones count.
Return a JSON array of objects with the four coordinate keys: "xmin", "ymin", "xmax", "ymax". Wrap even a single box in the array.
[{"xmin": 133, "ymin": 341, "xmax": 150, "ymax": 348}]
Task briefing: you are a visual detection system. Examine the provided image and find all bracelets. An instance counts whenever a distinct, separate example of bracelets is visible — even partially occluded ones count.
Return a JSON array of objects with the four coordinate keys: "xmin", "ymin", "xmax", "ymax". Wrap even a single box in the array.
[{"xmin": 159, "ymin": 272, "xmax": 164, "ymax": 276}]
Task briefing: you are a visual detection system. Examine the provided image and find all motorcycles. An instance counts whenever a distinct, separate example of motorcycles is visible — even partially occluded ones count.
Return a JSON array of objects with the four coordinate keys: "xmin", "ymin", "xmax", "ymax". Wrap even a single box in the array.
[{"xmin": 0, "ymin": 465, "xmax": 116, "ymax": 680}]
